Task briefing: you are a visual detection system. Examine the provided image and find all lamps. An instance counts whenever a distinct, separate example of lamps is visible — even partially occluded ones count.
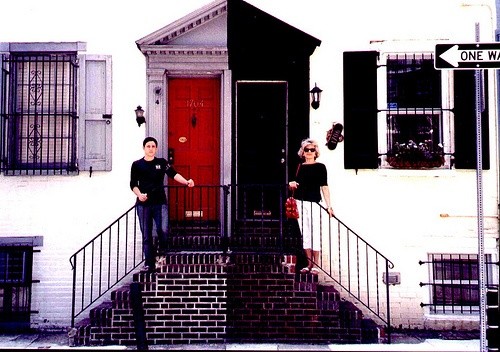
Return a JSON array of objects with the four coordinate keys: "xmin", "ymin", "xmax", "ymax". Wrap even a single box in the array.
[
  {"xmin": 134, "ymin": 104, "xmax": 146, "ymax": 127},
  {"xmin": 308, "ymin": 82, "xmax": 324, "ymax": 110}
]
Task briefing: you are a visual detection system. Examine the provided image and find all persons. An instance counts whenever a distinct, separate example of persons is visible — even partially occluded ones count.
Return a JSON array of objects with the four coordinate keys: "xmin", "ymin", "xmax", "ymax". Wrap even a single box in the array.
[
  {"xmin": 289, "ymin": 138, "xmax": 334, "ymax": 275},
  {"xmin": 130, "ymin": 137, "xmax": 194, "ymax": 273}
]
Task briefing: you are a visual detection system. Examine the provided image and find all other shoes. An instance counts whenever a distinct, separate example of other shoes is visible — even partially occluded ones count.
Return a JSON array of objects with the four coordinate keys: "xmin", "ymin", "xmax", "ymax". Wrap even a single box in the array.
[
  {"xmin": 140, "ymin": 265, "xmax": 150, "ymax": 272},
  {"xmin": 155, "ymin": 255, "xmax": 166, "ymax": 268}
]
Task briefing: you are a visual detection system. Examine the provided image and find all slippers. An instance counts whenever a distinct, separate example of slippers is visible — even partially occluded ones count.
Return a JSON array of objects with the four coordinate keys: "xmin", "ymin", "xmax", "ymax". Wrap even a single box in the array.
[
  {"xmin": 299, "ymin": 266, "xmax": 310, "ymax": 274},
  {"xmin": 310, "ymin": 268, "xmax": 319, "ymax": 275}
]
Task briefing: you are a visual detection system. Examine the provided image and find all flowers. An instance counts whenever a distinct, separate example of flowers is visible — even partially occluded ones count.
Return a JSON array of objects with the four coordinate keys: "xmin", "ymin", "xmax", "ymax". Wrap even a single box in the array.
[{"xmin": 385, "ymin": 139, "xmax": 445, "ymax": 164}]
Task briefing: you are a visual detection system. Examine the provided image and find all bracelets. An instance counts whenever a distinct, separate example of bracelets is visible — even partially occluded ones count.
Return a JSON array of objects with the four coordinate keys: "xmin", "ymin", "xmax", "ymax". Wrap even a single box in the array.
[{"xmin": 327, "ymin": 206, "xmax": 332, "ymax": 211}]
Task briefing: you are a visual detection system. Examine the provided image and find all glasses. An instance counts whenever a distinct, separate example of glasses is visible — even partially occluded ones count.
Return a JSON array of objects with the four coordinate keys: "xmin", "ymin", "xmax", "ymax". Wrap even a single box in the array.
[{"xmin": 304, "ymin": 147, "xmax": 316, "ymax": 152}]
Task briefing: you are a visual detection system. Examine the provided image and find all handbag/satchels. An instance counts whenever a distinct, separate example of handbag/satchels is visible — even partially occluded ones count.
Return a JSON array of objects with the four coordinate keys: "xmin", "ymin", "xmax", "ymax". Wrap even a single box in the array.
[{"xmin": 285, "ymin": 197, "xmax": 299, "ymax": 218}]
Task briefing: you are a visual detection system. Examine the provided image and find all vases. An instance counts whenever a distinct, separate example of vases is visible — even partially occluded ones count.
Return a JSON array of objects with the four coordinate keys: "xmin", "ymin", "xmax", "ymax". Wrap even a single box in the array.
[{"xmin": 385, "ymin": 153, "xmax": 445, "ymax": 168}]
